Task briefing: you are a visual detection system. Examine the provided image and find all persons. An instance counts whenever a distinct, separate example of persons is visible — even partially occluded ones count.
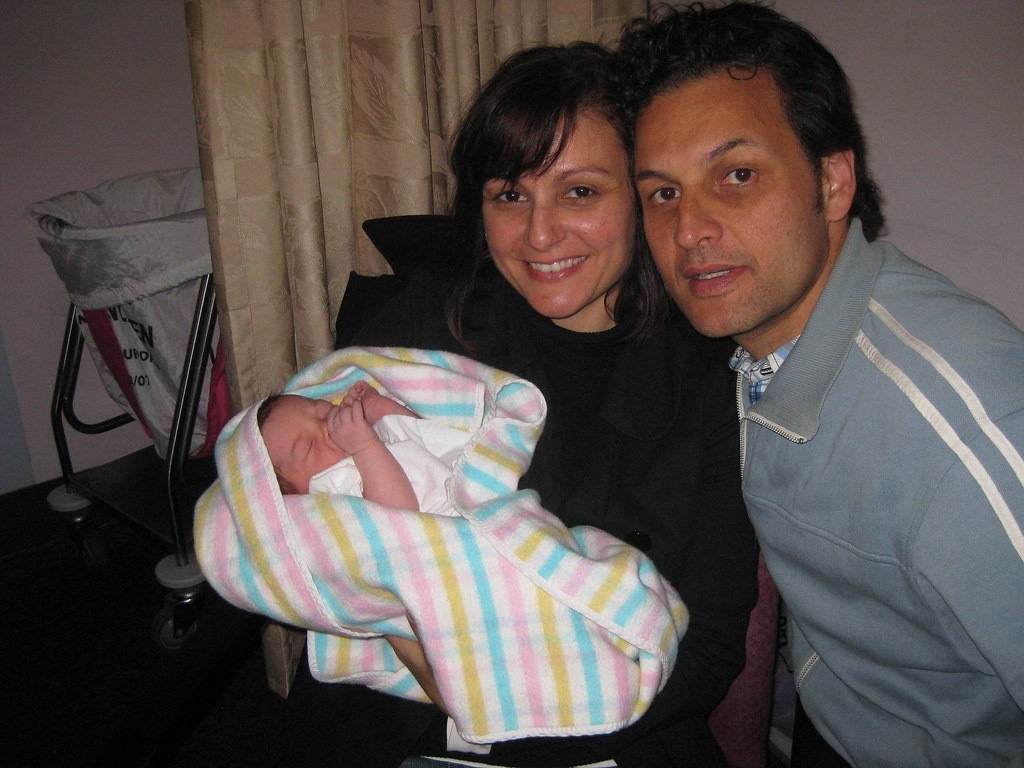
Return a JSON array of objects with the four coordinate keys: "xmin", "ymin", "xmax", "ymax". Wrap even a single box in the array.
[
  {"xmin": 610, "ymin": 1, "xmax": 1024, "ymax": 768},
  {"xmin": 255, "ymin": 377, "xmax": 694, "ymax": 743},
  {"xmin": 275, "ymin": 38, "xmax": 762, "ymax": 767}
]
